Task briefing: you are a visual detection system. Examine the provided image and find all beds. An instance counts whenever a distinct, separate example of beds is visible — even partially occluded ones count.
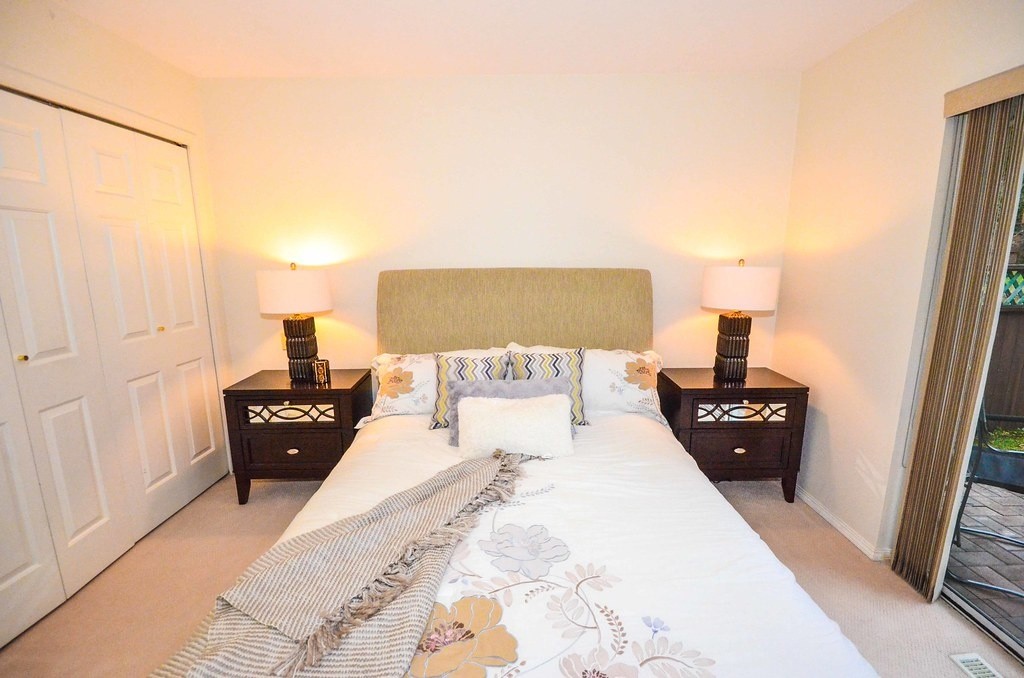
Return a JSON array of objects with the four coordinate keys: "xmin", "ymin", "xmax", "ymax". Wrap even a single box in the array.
[{"xmin": 145, "ymin": 269, "xmax": 887, "ymax": 678}]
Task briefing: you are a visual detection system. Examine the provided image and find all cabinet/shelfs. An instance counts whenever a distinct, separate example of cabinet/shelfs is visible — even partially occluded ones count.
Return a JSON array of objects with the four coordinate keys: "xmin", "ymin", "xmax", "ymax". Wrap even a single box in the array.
[{"xmin": 1, "ymin": 86, "xmax": 231, "ymax": 654}]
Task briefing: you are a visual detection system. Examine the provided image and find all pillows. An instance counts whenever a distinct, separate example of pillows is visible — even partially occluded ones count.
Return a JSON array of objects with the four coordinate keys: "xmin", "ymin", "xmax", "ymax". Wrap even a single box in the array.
[
  {"xmin": 430, "ymin": 350, "xmax": 588, "ymax": 432},
  {"xmin": 445, "ymin": 380, "xmax": 578, "ymax": 444},
  {"xmin": 455, "ymin": 395, "xmax": 575, "ymax": 460},
  {"xmin": 352, "ymin": 350, "xmax": 518, "ymax": 430},
  {"xmin": 505, "ymin": 342, "xmax": 672, "ymax": 433}
]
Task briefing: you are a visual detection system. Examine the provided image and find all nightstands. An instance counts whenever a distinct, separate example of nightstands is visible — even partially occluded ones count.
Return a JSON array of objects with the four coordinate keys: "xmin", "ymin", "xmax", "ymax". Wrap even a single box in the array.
[
  {"xmin": 220, "ymin": 369, "xmax": 374, "ymax": 505},
  {"xmin": 656, "ymin": 365, "xmax": 810, "ymax": 502}
]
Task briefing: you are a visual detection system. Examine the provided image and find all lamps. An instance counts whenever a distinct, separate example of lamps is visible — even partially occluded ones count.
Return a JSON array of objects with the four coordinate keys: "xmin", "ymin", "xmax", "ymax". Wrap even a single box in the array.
[
  {"xmin": 255, "ymin": 264, "xmax": 335, "ymax": 383},
  {"xmin": 700, "ymin": 260, "xmax": 780, "ymax": 382}
]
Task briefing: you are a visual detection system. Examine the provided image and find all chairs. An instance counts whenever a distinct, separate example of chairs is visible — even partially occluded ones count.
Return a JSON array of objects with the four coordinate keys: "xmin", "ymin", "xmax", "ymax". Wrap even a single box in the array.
[{"xmin": 947, "ymin": 399, "xmax": 1024, "ymax": 597}]
